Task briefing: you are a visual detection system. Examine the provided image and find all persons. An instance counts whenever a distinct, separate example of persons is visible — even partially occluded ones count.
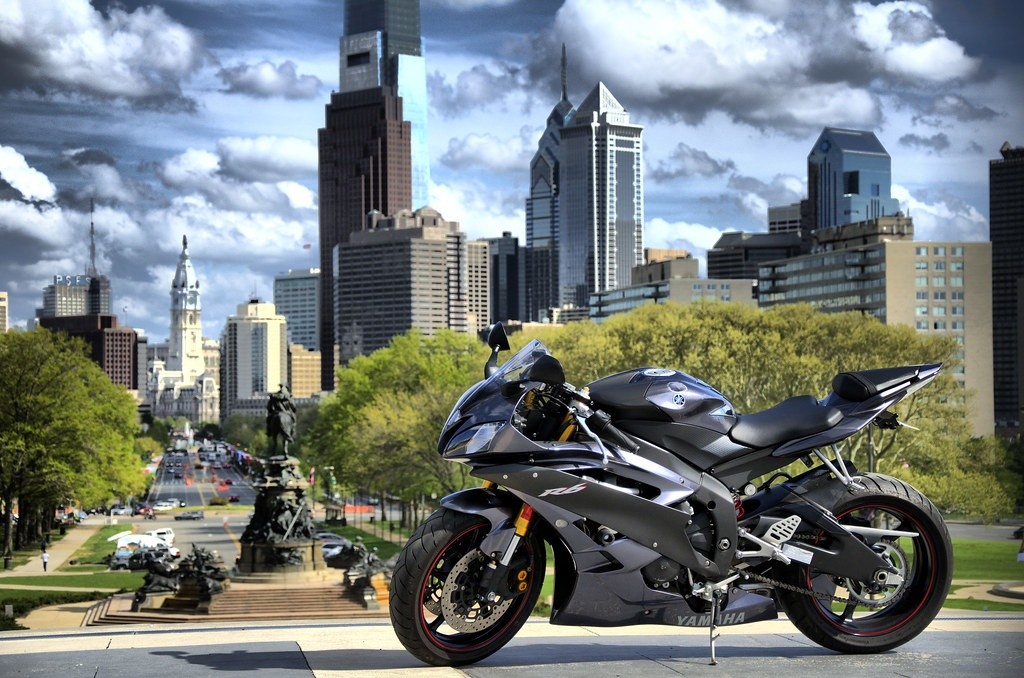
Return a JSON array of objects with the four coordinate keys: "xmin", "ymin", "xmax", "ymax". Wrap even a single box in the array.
[
  {"xmin": 41, "ymin": 549, "xmax": 49, "ymax": 569},
  {"xmin": 41, "ymin": 538, "xmax": 46, "ymax": 553},
  {"xmin": 267, "ymin": 383, "xmax": 297, "ymax": 438},
  {"xmin": 348, "ymin": 536, "xmax": 384, "ymax": 572}
]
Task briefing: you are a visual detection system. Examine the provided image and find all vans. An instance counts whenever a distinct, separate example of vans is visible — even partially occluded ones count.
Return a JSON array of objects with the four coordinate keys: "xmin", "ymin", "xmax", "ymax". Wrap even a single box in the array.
[
  {"xmin": 144, "ymin": 528, "xmax": 175, "ymax": 544},
  {"xmin": 107, "ymin": 531, "xmax": 180, "ymax": 560}
]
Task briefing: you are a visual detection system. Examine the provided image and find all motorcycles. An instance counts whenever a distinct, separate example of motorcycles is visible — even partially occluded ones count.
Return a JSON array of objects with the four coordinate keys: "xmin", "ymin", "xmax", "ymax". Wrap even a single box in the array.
[{"xmin": 388, "ymin": 321, "xmax": 954, "ymax": 667}]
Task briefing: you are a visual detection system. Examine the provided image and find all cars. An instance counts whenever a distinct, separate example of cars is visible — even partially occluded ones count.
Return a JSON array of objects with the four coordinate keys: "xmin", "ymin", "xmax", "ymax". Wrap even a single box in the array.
[
  {"xmin": 84, "ymin": 440, "xmax": 255, "ymax": 515},
  {"xmin": 368, "ymin": 496, "xmax": 378, "ymax": 505},
  {"xmin": 316, "ymin": 529, "xmax": 355, "ymax": 557}
]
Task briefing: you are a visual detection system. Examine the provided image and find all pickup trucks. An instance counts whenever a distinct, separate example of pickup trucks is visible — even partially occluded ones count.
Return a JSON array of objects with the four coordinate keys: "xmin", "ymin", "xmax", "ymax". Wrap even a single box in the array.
[
  {"xmin": 174, "ymin": 511, "xmax": 203, "ymax": 520},
  {"xmin": 110, "ymin": 549, "xmax": 180, "ymax": 573}
]
